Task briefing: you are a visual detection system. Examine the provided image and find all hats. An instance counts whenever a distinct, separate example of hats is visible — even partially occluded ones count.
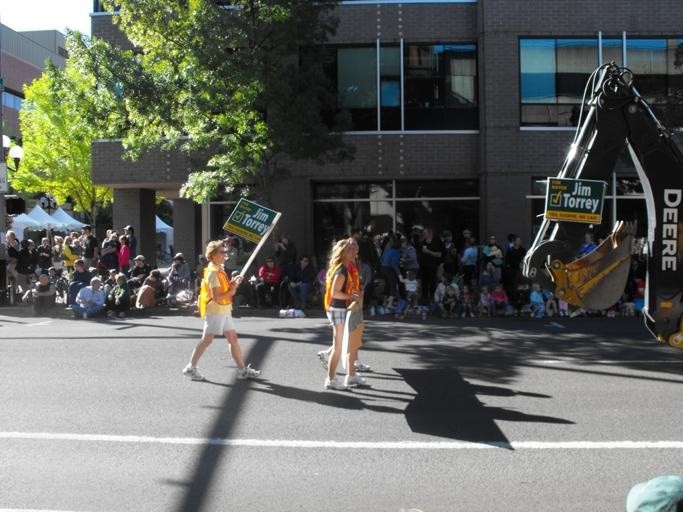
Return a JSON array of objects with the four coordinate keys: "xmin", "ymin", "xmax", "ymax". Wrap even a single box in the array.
[
  {"xmin": 133, "ymin": 255, "xmax": 144, "ymax": 261},
  {"xmin": 123, "ymin": 225, "xmax": 133, "ymax": 231}
]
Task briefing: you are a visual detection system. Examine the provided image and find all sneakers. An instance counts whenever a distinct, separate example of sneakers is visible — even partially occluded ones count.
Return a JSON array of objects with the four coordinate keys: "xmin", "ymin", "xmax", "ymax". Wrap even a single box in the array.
[
  {"xmin": 182, "ymin": 364, "xmax": 204, "ymax": 381},
  {"xmin": 353, "ymin": 362, "xmax": 372, "ymax": 372},
  {"xmin": 345, "ymin": 375, "xmax": 366, "ymax": 386},
  {"xmin": 325, "ymin": 378, "xmax": 348, "ymax": 391},
  {"xmin": 316, "ymin": 351, "xmax": 329, "ymax": 370},
  {"xmin": 236, "ymin": 367, "xmax": 261, "ymax": 379}
]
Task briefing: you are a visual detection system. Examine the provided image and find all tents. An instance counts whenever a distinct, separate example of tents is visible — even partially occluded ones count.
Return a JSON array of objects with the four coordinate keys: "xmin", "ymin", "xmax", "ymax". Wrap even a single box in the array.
[
  {"xmin": 13, "ymin": 205, "xmax": 85, "ymax": 231},
  {"xmin": 154, "ymin": 215, "xmax": 173, "ymax": 253}
]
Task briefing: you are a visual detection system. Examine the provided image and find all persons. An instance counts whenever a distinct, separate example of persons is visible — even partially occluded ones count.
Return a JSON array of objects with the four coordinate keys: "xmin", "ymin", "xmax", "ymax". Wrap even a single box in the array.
[
  {"xmin": 223, "ymin": 232, "xmax": 327, "ymax": 313},
  {"xmin": 322, "ymin": 240, "xmax": 372, "ymax": 390},
  {"xmin": 1, "ymin": 225, "xmax": 191, "ymax": 319},
  {"xmin": 181, "ymin": 238, "xmax": 261, "ymax": 381},
  {"xmin": 317, "ymin": 237, "xmax": 374, "ymax": 374},
  {"xmin": 351, "ymin": 223, "xmax": 646, "ymax": 319}
]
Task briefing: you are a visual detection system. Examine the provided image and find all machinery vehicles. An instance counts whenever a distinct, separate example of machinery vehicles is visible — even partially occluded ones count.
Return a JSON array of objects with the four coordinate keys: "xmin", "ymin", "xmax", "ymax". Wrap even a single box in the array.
[{"xmin": 520, "ymin": 60, "xmax": 682, "ymax": 352}]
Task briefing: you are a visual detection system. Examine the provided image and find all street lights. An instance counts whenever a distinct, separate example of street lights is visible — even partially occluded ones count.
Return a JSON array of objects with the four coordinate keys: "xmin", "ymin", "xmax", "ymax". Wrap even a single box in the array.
[
  {"xmin": 3, "ymin": 135, "xmax": 24, "ymax": 173},
  {"xmin": 40, "ymin": 191, "xmax": 58, "ymax": 247}
]
